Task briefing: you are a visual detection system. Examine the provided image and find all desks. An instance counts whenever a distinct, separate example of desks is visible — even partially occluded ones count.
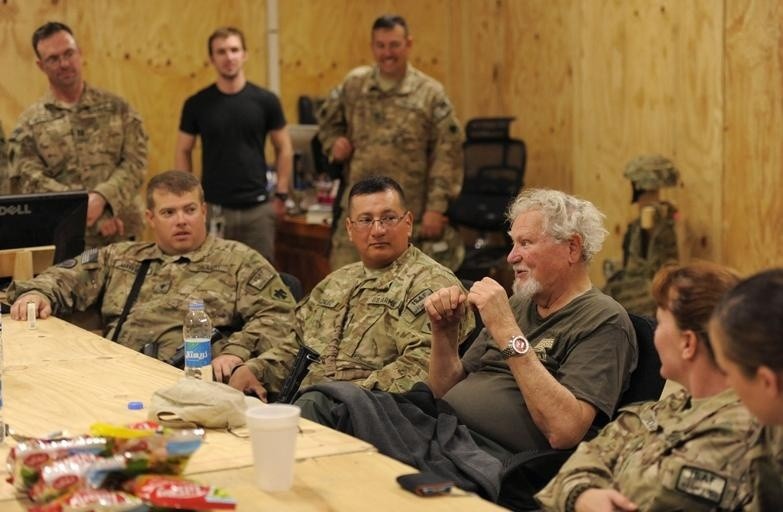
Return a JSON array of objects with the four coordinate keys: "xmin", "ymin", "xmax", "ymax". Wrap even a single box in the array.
[{"xmin": 274, "ymin": 190, "xmax": 331, "ymax": 302}]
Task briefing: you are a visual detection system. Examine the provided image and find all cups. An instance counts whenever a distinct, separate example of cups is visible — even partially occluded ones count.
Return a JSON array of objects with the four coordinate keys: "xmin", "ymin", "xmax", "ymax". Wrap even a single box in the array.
[{"xmin": 245, "ymin": 404, "xmax": 300, "ymax": 493}]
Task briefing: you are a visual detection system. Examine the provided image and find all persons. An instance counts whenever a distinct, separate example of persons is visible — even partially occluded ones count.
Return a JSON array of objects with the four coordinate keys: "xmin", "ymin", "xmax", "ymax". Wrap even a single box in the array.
[
  {"xmin": 310, "ymin": 12, "xmax": 468, "ymax": 271},
  {"xmin": 172, "ymin": 23, "xmax": 296, "ymax": 267},
  {"xmin": 0, "ymin": 169, "xmax": 303, "ymax": 387},
  {"xmin": 703, "ymin": 263, "xmax": 782, "ymax": 512},
  {"xmin": 296, "ymin": 184, "xmax": 644, "ymax": 512},
  {"xmin": 2, "ymin": 19, "xmax": 153, "ymax": 250},
  {"xmin": 526, "ymin": 257, "xmax": 766, "ymax": 511},
  {"xmin": 226, "ymin": 172, "xmax": 480, "ymax": 405}
]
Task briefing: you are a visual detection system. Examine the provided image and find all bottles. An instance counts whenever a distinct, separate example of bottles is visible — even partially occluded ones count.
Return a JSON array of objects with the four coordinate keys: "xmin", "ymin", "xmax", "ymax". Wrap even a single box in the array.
[
  {"xmin": 121, "ymin": 402, "xmax": 147, "ymax": 424},
  {"xmin": 182, "ymin": 298, "xmax": 213, "ymax": 384}
]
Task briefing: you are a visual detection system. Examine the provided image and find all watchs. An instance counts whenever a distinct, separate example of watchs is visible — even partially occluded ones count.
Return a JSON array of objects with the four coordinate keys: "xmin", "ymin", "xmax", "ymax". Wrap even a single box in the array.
[{"xmin": 500, "ymin": 334, "xmax": 529, "ymax": 361}]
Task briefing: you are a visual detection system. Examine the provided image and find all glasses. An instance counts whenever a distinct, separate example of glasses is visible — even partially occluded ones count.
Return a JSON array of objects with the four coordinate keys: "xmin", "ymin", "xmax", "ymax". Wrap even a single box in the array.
[{"xmin": 349, "ymin": 211, "xmax": 408, "ymax": 227}]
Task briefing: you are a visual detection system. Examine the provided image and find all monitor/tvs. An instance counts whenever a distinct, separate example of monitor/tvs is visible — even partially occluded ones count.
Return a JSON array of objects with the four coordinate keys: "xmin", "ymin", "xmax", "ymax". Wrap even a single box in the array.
[
  {"xmin": 286, "ymin": 125, "xmax": 322, "ymax": 191},
  {"xmin": 0, "ymin": 190, "xmax": 90, "ymax": 291}
]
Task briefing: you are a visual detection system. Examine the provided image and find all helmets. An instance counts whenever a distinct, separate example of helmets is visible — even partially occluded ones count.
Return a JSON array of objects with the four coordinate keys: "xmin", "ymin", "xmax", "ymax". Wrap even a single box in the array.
[{"xmin": 624, "ymin": 154, "xmax": 679, "ymax": 204}]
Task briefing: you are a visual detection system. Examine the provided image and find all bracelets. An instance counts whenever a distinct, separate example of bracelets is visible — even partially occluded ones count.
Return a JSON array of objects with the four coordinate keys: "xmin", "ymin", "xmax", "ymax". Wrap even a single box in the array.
[{"xmin": 272, "ymin": 191, "xmax": 288, "ymax": 202}]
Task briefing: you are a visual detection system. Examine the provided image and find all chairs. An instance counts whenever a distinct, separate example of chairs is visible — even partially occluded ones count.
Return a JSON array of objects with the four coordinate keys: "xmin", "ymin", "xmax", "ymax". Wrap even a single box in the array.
[
  {"xmin": 444, "ymin": 116, "xmax": 527, "ymax": 281},
  {"xmin": 458, "ymin": 314, "xmax": 665, "ymax": 512}
]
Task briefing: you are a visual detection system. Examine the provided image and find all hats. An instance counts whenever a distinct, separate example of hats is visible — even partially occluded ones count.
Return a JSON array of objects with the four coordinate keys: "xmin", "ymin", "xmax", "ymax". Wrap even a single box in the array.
[
  {"xmin": 412, "ymin": 221, "xmax": 466, "ymax": 274},
  {"xmin": 148, "ymin": 377, "xmax": 250, "ymax": 439}
]
